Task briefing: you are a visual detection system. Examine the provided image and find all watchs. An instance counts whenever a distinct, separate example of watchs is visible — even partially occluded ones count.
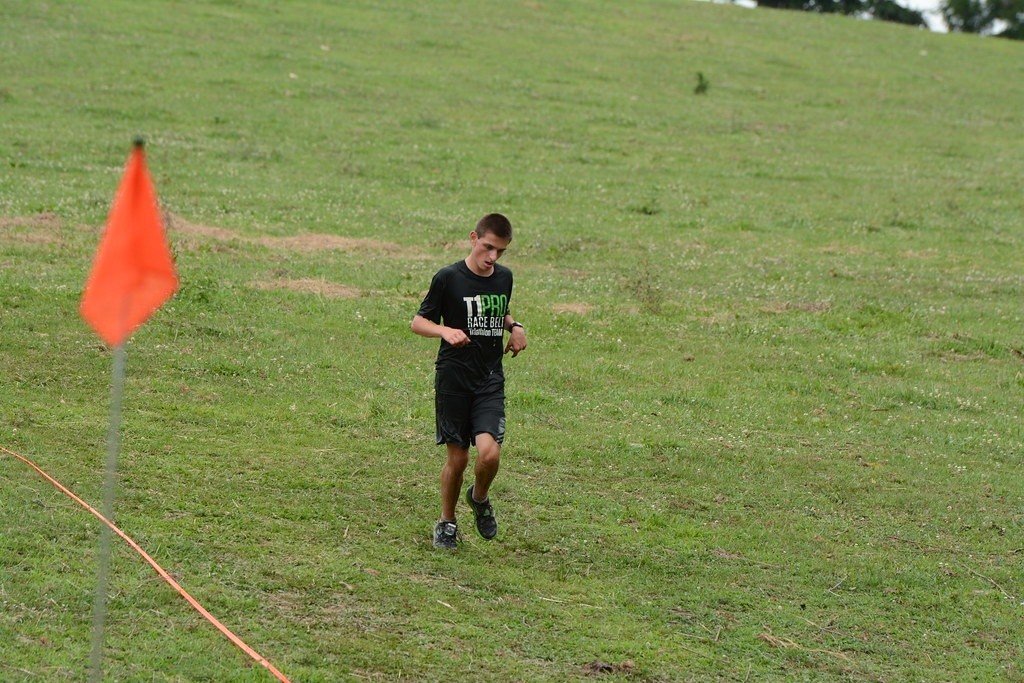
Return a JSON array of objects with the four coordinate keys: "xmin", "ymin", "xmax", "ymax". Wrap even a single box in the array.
[{"xmin": 509, "ymin": 321, "xmax": 523, "ymax": 334}]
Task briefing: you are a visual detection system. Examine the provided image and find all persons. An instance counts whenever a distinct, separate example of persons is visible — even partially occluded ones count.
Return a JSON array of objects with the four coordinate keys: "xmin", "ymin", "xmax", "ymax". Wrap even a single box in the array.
[{"xmin": 410, "ymin": 213, "xmax": 529, "ymax": 551}]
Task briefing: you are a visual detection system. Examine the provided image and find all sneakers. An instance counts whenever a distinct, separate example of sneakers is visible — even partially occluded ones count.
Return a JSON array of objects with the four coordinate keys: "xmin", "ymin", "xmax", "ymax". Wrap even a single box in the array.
[
  {"xmin": 466, "ymin": 485, "xmax": 497, "ymax": 540},
  {"xmin": 433, "ymin": 519, "xmax": 457, "ymax": 552}
]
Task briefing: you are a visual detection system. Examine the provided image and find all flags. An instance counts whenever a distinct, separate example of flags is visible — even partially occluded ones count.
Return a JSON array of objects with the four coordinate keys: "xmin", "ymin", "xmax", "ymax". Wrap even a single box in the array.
[{"xmin": 83, "ymin": 148, "xmax": 176, "ymax": 348}]
